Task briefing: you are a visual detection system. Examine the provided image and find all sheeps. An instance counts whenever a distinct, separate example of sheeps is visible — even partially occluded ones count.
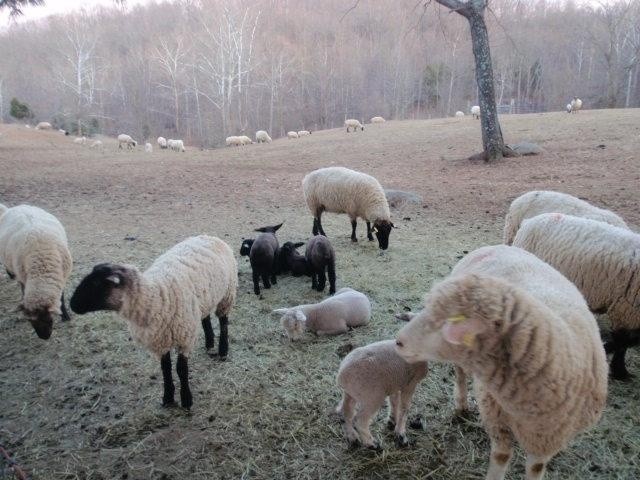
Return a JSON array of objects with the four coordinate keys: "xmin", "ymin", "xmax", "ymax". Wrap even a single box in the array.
[
  {"xmin": 566, "ymin": 96, "xmax": 582, "ymax": 113},
  {"xmin": 237, "ymin": 222, "xmax": 371, "ymax": 343},
  {"xmin": 225, "ymin": 130, "xmax": 273, "ymax": 146},
  {"xmin": 302, "ymin": 167, "xmax": 394, "ymax": 249},
  {"xmin": 455, "ymin": 106, "xmax": 481, "ymax": 118},
  {"xmin": 69, "ymin": 234, "xmax": 240, "ymax": 410},
  {"xmin": 394, "ymin": 189, "xmax": 640, "ymax": 480},
  {"xmin": 345, "ymin": 119, "xmax": 365, "ymax": 133},
  {"xmin": 286, "ymin": 130, "xmax": 311, "ymax": 139},
  {"xmin": 1, "ymin": 202, "xmax": 70, "ymax": 339},
  {"xmin": 335, "ymin": 311, "xmax": 428, "ymax": 448},
  {"xmin": 24, "ymin": 120, "xmax": 101, "ymax": 149},
  {"xmin": 371, "ymin": 117, "xmax": 385, "ymax": 124},
  {"xmin": 117, "ymin": 132, "xmax": 186, "ymax": 154}
]
